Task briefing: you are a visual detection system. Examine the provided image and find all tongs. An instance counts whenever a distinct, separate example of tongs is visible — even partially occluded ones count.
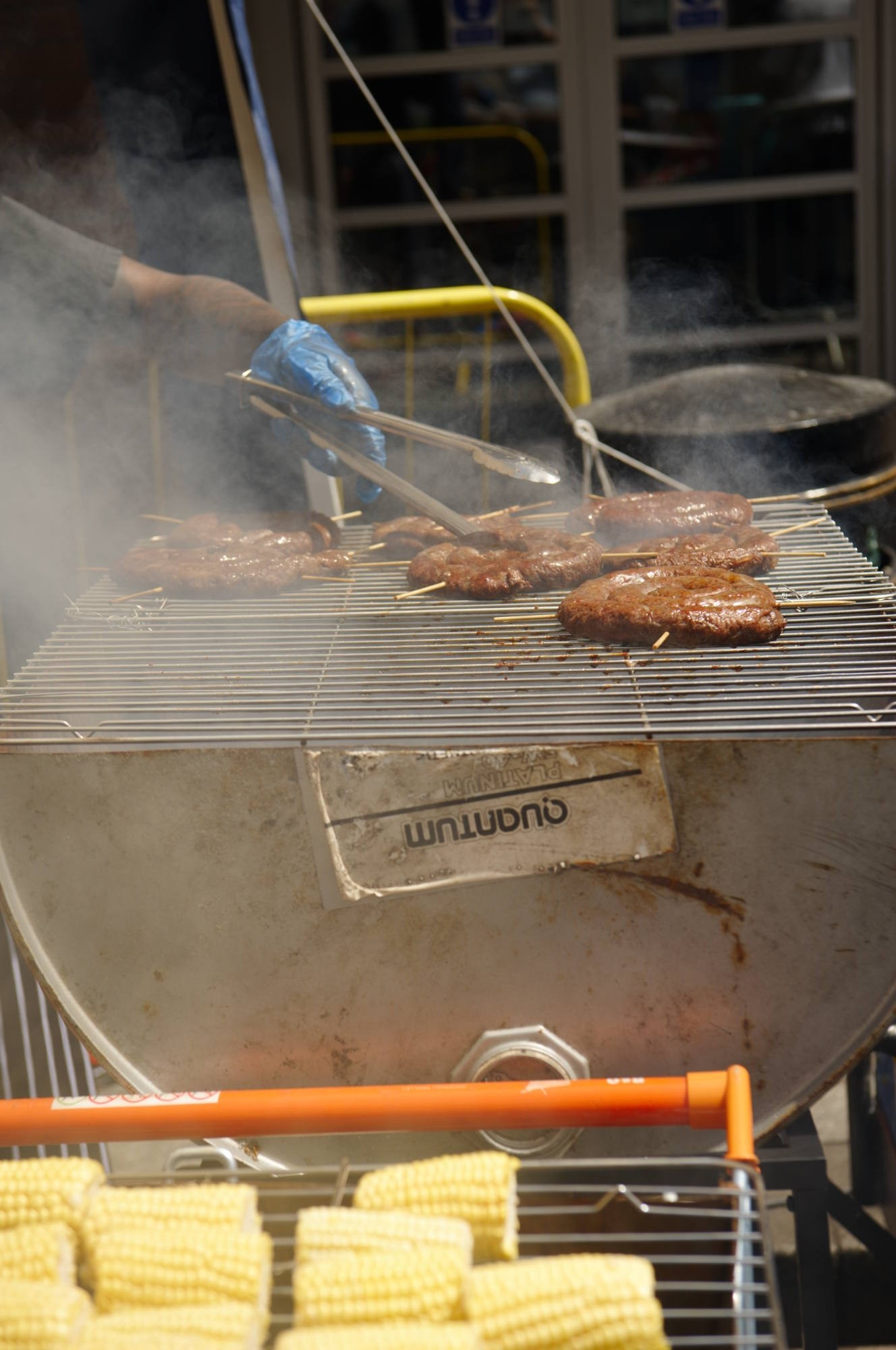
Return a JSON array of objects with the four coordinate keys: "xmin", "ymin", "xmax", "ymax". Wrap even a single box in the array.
[{"xmin": 225, "ymin": 371, "xmax": 561, "ymax": 553}]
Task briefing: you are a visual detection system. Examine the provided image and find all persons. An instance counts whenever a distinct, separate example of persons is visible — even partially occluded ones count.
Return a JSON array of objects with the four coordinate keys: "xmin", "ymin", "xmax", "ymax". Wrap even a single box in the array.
[{"xmin": 0, "ymin": 192, "xmax": 385, "ymax": 1174}]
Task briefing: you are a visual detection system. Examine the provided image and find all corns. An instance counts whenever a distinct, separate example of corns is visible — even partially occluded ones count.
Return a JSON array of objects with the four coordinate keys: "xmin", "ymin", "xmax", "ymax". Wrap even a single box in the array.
[{"xmin": 0, "ymin": 1148, "xmax": 673, "ymax": 1350}]
